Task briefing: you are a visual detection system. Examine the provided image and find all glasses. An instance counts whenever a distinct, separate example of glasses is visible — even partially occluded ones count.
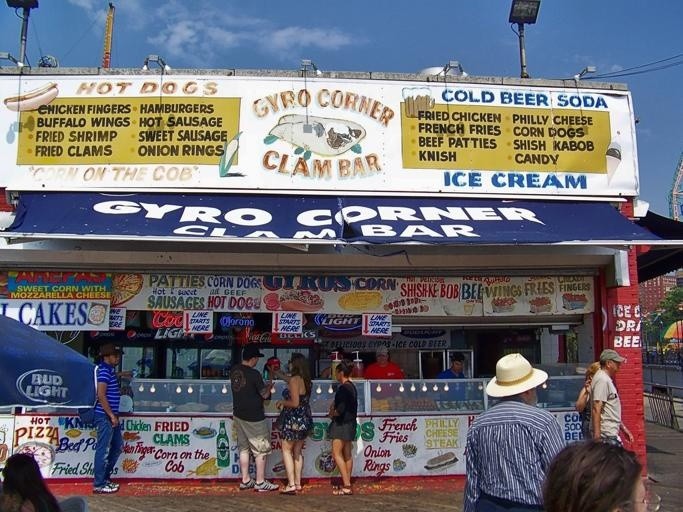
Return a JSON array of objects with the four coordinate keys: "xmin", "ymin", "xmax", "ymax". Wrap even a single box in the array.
[
  {"xmin": 633, "ymin": 489, "xmax": 661, "ymax": 512},
  {"xmin": 287, "ymin": 360, "xmax": 293, "ymax": 364}
]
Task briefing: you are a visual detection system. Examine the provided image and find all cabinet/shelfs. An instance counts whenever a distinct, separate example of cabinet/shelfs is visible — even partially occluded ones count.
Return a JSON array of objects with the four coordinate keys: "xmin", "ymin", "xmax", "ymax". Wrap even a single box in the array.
[{"xmin": 0, "ymin": 375, "xmax": 593, "ymax": 494}]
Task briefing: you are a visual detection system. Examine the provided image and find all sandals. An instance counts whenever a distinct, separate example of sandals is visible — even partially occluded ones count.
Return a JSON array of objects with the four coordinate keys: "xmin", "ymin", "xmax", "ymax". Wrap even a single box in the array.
[
  {"xmin": 331, "ymin": 482, "xmax": 345, "ymax": 489},
  {"xmin": 280, "ymin": 483, "xmax": 296, "ymax": 495},
  {"xmin": 295, "ymin": 483, "xmax": 301, "ymax": 492},
  {"xmin": 332, "ymin": 485, "xmax": 352, "ymax": 496}
]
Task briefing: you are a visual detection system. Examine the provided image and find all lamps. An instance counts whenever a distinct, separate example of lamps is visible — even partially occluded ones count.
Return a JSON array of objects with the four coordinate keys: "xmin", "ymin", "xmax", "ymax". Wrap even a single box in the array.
[
  {"xmin": 508, "ymin": 0, "xmax": 540, "ymax": 79},
  {"xmin": 6, "ymin": 0, "xmax": 39, "ymax": 62}
]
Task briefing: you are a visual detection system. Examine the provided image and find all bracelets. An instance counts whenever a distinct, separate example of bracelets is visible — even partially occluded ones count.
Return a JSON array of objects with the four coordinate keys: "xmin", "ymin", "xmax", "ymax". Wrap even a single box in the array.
[{"xmin": 621, "ymin": 425, "xmax": 627, "ymax": 432}]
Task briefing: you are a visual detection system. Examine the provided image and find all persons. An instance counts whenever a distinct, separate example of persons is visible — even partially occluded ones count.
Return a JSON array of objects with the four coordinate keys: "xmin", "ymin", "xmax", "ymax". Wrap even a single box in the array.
[
  {"xmin": 433, "ymin": 351, "xmax": 467, "ymax": 401},
  {"xmin": 0, "ymin": 452, "xmax": 62, "ymax": 512},
  {"xmin": 229, "ymin": 341, "xmax": 406, "ymax": 495},
  {"xmin": 590, "ymin": 348, "xmax": 634, "ymax": 446},
  {"xmin": 92, "ymin": 343, "xmax": 134, "ymax": 493},
  {"xmin": 542, "ymin": 436, "xmax": 662, "ymax": 512},
  {"xmin": 575, "ymin": 361, "xmax": 601, "ymax": 439},
  {"xmin": 462, "ymin": 352, "xmax": 565, "ymax": 511}
]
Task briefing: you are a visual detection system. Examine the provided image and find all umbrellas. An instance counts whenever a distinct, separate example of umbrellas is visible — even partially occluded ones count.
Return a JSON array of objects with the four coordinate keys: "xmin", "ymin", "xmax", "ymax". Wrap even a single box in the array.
[{"xmin": 0, "ymin": 313, "xmax": 98, "ymax": 456}]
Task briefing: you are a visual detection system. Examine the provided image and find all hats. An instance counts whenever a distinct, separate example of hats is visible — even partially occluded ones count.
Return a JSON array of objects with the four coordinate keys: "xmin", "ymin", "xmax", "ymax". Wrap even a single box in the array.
[
  {"xmin": 242, "ymin": 343, "xmax": 264, "ymax": 360},
  {"xmin": 484, "ymin": 353, "xmax": 548, "ymax": 398},
  {"xmin": 599, "ymin": 349, "xmax": 624, "ymax": 363},
  {"xmin": 97, "ymin": 342, "xmax": 124, "ymax": 356},
  {"xmin": 376, "ymin": 345, "xmax": 389, "ymax": 356},
  {"xmin": 450, "ymin": 352, "xmax": 465, "ymax": 361}
]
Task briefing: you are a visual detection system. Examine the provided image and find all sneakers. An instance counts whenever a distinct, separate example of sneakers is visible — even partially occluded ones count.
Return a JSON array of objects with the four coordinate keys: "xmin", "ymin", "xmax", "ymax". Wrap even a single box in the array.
[
  {"xmin": 254, "ymin": 480, "xmax": 279, "ymax": 491},
  {"xmin": 239, "ymin": 478, "xmax": 254, "ymax": 490},
  {"xmin": 94, "ymin": 485, "xmax": 118, "ymax": 493},
  {"xmin": 107, "ymin": 483, "xmax": 119, "ymax": 488}
]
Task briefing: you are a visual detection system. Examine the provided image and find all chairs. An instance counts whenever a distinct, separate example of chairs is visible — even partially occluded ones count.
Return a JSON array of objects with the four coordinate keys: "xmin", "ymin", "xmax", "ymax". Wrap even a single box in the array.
[{"xmin": 56, "ymin": 496, "xmax": 87, "ymax": 512}]
